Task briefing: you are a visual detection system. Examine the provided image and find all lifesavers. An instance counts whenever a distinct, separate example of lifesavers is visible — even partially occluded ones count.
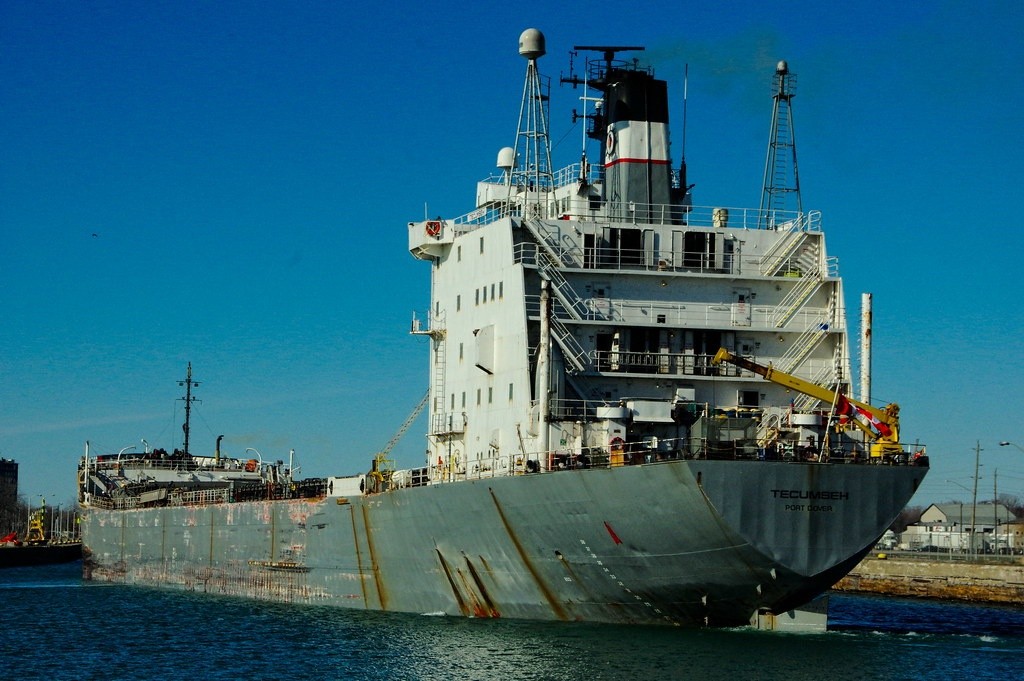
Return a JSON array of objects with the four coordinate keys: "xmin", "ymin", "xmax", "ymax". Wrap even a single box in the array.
[
  {"xmin": 611, "ymin": 437, "xmax": 624, "ymax": 450},
  {"xmin": 426, "ymin": 221, "xmax": 440, "ymax": 235}
]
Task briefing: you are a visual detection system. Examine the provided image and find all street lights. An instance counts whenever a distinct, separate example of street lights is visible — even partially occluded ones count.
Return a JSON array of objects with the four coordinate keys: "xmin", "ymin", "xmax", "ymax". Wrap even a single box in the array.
[{"xmin": 38, "ymin": 493, "xmax": 76, "ymax": 544}]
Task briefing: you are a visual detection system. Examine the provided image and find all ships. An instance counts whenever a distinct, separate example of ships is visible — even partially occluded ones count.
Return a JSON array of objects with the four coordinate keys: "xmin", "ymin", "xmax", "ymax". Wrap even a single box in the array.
[{"xmin": 77, "ymin": 27, "xmax": 933, "ymax": 637}]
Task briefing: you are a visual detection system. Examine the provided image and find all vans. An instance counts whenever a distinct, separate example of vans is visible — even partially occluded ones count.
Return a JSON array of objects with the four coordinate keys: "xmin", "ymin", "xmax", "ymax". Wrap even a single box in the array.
[{"xmin": 882, "ymin": 535, "xmax": 897, "ymax": 549}]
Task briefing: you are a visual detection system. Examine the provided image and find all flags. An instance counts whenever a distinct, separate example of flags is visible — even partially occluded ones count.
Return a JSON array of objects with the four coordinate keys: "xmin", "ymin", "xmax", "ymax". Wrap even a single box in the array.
[{"xmin": 836, "ymin": 384, "xmax": 894, "ymax": 437}]
